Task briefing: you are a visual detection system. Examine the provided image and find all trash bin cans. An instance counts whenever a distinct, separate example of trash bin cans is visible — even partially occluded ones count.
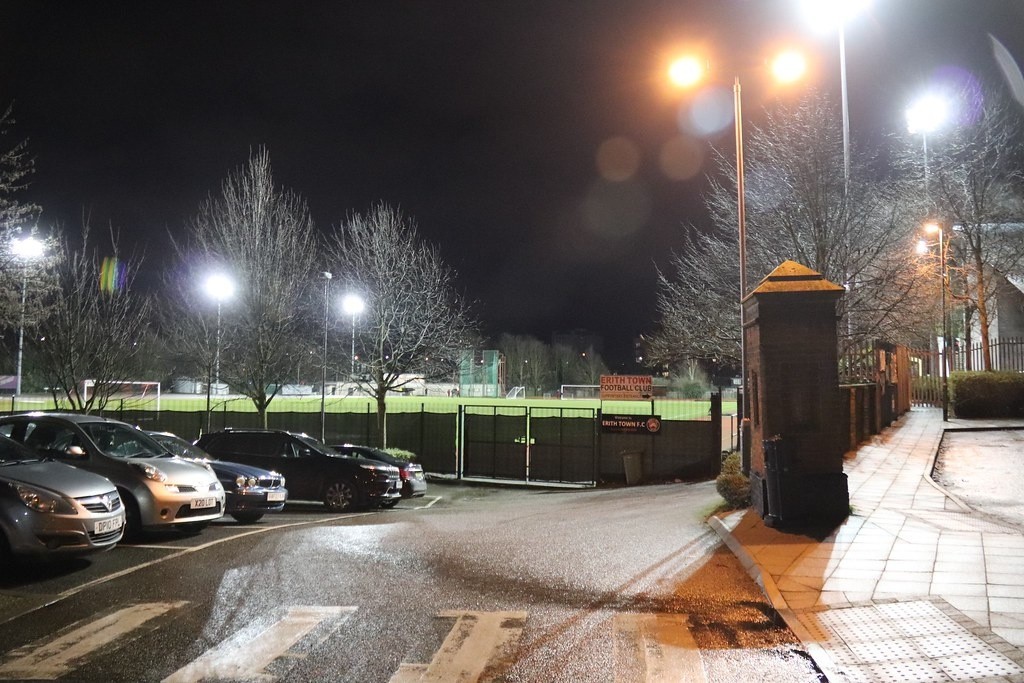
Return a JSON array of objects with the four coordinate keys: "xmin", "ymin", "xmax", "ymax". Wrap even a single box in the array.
[{"xmin": 620, "ymin": 449, "xmax": 645, "ymax": 487}]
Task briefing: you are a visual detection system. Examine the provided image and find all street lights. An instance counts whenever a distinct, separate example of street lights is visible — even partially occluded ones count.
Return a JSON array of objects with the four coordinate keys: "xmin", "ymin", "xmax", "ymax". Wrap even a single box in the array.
[
  {"xmin": 5, "ymin": 236, "xmax": 47, "ymax": 396},
  {"xmin": 904, "ymin": 89, "xmax": 943, "ymax": 221},
  {"xmin": 200, "ymin": 264, "xmax": 235, "ymax": 398},
  {"xmin": 664, "ymin": 47, "xmax": 814, "ymax": 481},
  {"xmin": 340, "ymin": 288, "xmax": 364, "ymax": 395},
  {"xmin": 912, "ymin": 223, "xmax": 950, "ymax": 423}
]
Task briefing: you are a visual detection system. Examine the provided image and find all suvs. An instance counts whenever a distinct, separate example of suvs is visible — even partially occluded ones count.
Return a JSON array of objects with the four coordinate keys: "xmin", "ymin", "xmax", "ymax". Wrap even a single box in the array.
[{"xmin": 191, "ymin": 426, "xmax": 403, "ymax": 509}]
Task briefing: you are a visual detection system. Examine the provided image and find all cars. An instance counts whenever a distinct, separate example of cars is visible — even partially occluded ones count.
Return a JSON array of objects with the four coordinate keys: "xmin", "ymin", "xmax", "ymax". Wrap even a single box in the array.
[
  {"xmin": 327, "ymin": 438, "xmax": 427, "ymax": 504},
  {"xmin": 0, "ymin": 430, "xmax": 126, "ymax": 563},
  {"xmin": 105, "ymin": 430, "xmax": 290, "ymax": 522},
  {"xmin": 0, "ymin": 411, "xmax": 225, "ymax": 539}
]
